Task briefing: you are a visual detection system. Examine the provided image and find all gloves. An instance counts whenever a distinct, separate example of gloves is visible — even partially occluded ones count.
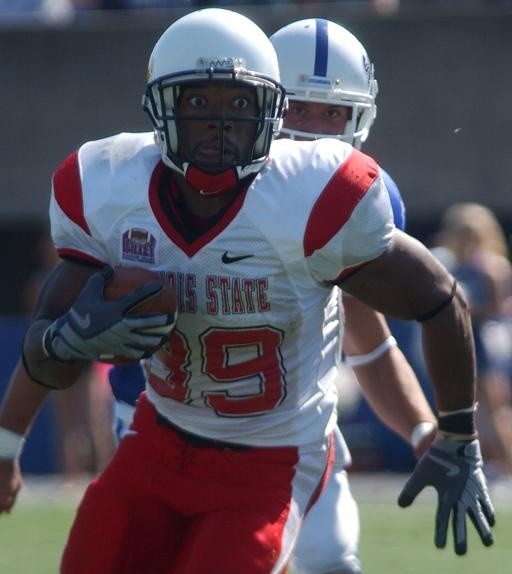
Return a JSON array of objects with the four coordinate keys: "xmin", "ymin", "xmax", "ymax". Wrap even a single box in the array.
[
  {"xmin": 40, "ymin": 264, "xmax": 175, "ymax": 361},
  {"xmin": 397, "ymin": 402, "xmax": 497, "ymax": 554}
]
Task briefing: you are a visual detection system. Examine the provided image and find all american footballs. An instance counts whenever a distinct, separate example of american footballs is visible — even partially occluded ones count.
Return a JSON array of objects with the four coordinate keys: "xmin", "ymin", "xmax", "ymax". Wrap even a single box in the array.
[{"xmin": 96, "ymin": 268, "xmax": 178, "ymax": 363}]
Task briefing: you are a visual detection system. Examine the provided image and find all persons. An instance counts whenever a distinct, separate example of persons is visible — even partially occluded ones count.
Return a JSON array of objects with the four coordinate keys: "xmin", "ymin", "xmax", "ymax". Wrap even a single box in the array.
[
  {"xmin": 29, "ymin": 232, "xmax": 116, "ymax": 473},
  {"xmin": 426, "ymin": 201, "xmax": 511, "ymax": 481},
  {"xmin": 1, "ymin": 17, "xmax": 439, "ymax": 574},
  {"xmin": 22, "ymin": 6, "xmax": 495, "ymax": 574}
]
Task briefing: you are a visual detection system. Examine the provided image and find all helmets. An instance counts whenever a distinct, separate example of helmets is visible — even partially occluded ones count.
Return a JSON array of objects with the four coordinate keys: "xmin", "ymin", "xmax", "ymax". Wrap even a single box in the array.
[
  {"xmin": 267, "ymin": 18, "xmax": 379, "ymax": 153},
  {"xmin": 142, "ymin": 8, "xmax": 286, "ymax": 198}
]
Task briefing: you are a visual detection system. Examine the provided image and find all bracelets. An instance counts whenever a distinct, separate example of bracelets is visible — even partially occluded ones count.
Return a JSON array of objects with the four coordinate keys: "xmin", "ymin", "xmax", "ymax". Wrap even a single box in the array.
[{"xmin": 0, "ymin": 429, "xmax": 24, "ymax": 457}]
